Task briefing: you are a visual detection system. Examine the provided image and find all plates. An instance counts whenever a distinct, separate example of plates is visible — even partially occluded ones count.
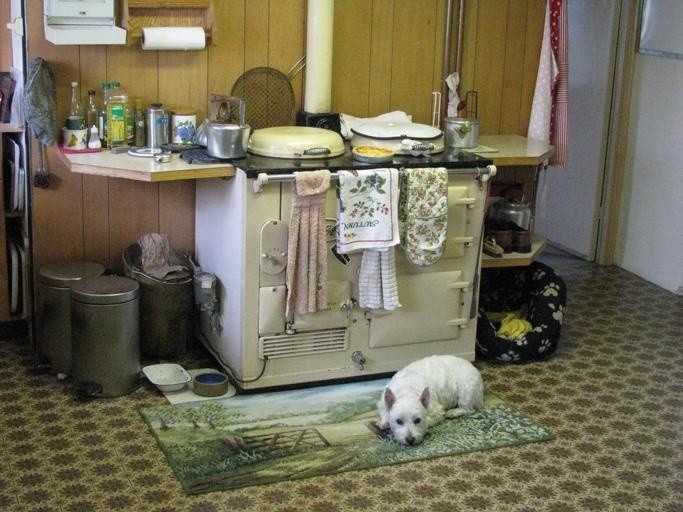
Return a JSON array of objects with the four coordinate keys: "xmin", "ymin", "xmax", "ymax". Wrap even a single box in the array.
[{"xmin": 142, "ymin": 362, "xmax": 193, "ymax": 393}]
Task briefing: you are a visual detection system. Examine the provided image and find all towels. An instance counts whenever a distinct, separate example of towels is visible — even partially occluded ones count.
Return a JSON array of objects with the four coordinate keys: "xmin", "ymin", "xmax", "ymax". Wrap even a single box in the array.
[
  {"xmin": 401, "ymin": 167, "xmax": 447, "ymax": 262},
  {"xmin": 337, "ymin": 168, "xmax": 400, "ymax": 250},
  {"xmin": 284, "ymin": 170, "xmax": 331, "ymax": 319},
  {"xmin": 359, "ymin": 249, "xmax": 400, "ymax": 312}
]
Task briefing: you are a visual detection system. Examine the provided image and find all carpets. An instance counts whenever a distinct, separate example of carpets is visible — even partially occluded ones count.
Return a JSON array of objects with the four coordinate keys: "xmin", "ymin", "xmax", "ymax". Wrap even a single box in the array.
[{"xmin": 128, "ymin": 372, "xmax": 554, "ymax": 497}]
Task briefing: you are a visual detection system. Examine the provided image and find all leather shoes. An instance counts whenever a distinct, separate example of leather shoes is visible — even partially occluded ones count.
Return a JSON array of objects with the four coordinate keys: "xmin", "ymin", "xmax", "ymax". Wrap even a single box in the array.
[{"xmin": 487, "ymin": 219, "xmax": 531, "ymax": 253}]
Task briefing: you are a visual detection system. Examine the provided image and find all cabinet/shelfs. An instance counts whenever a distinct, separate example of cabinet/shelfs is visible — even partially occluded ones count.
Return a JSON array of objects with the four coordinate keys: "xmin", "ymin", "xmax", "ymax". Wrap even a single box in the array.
[{"xmin": 0, "ymin": 124, "xmax": 33, "ymax": 336}]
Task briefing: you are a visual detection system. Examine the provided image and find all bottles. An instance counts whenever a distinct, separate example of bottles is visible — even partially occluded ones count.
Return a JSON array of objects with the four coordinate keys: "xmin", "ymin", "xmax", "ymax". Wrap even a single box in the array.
[
  {"xmin": 145, "ymin": 103, "xmax": 168, "ymax": 148},
  {"xmin": 170, "ymin": 109, "xmax": 196, "ymax": 146},
  {"xmin": 66, "ymin": 81, "xmax": 144, "ymax": 149}
]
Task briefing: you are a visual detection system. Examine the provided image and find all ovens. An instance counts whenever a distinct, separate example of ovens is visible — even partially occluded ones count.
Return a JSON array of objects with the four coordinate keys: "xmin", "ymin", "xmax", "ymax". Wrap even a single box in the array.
[{"xmin": 242, "ymin": 164, "xmax": 497, "ymax": 392}]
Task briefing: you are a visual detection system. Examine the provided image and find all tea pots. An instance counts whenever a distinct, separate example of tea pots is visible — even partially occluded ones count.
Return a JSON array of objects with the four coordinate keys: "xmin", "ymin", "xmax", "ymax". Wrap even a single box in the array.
[
  {"xmin": 203, "ymin": 91, "xmax": 252, "ymax": 160},
  {"xmin": 495, "ymin": 182, "xmax": 533, "ymax": 231}
]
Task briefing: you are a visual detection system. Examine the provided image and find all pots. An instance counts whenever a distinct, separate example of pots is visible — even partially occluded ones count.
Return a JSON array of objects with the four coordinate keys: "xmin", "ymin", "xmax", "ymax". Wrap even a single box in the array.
[
  {"xmin": 350, "ymin": 121, "xmax": 446, "ymax": 159},
  {"xmin": 248, "ymin": 125, "xmax": 344, "ymax": 159}
]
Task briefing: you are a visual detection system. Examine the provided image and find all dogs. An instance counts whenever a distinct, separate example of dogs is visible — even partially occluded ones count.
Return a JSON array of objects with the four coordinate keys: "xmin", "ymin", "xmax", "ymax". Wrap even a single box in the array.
[{"xmin": 376, "ymin": 354, "xmax": 486, "ymax": 446}]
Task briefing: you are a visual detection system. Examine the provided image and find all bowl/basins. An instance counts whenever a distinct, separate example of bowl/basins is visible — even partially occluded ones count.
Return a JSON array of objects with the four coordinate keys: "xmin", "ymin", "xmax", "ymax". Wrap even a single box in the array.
[
  {"xmin": 192, "ymin": 372, "xmax": 230, "ymax": 397},
  {"xmin": 444, "ymin": 118, "xmax": 480, "ymax": 149}
]
